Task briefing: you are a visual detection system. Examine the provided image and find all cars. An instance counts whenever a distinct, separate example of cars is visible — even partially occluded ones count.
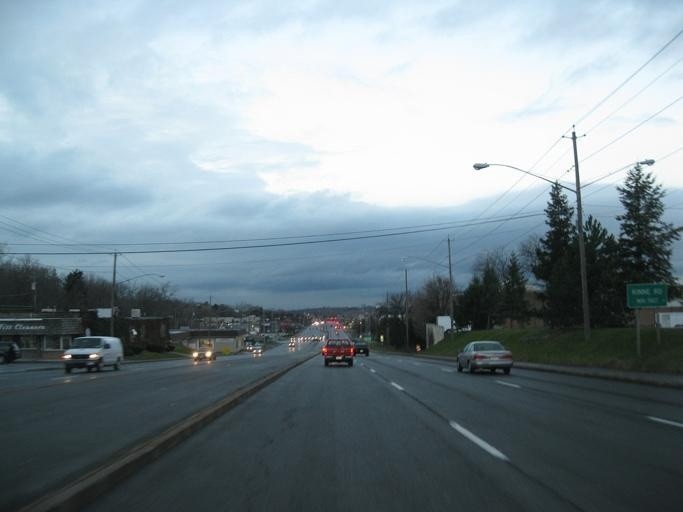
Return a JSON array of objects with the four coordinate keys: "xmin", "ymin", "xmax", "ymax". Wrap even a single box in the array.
[
  {"xmin": 246, "ymin": 344, "xmax": 263, "ymax": 354},
  {"xmin": 193, "ymin": 346, "xmax": 216, "ymax": 361},
  {"xmin": 0, "ymin": 340, "xmax": 21, "ymax": 364},
  {"xmin": 352, "ymin": 337, "xmax": 369, "ymax": 357},
  {"xmin": 457, "ymin": 340, "xmax": 514, "ymax": 375}
]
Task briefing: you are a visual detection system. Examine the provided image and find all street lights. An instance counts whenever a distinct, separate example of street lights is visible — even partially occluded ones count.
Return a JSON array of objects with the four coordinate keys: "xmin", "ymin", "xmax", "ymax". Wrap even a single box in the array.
[
  {"xmin": 473, "ymin": 159, "xmax": 655, "ymax": 343},
  {"xmin": 110, "ymin": 273, "xmax": 165, "ymax": 337},
  {"xmin": 399, "ymin": 255, "xmax": 454, "ymax": 345}
]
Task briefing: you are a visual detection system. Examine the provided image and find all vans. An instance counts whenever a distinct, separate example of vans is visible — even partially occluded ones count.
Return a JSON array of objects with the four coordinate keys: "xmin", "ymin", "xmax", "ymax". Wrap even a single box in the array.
[{"xmin": 61, "ymin": 336, "xmax": 124, "ymax": 373}]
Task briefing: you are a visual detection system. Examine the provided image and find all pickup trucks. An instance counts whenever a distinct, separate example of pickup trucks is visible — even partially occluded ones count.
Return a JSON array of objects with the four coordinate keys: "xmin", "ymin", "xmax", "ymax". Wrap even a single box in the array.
[{"xmin": 322, "ymin": 338, "xmax": 354, "ymax": 367}]
